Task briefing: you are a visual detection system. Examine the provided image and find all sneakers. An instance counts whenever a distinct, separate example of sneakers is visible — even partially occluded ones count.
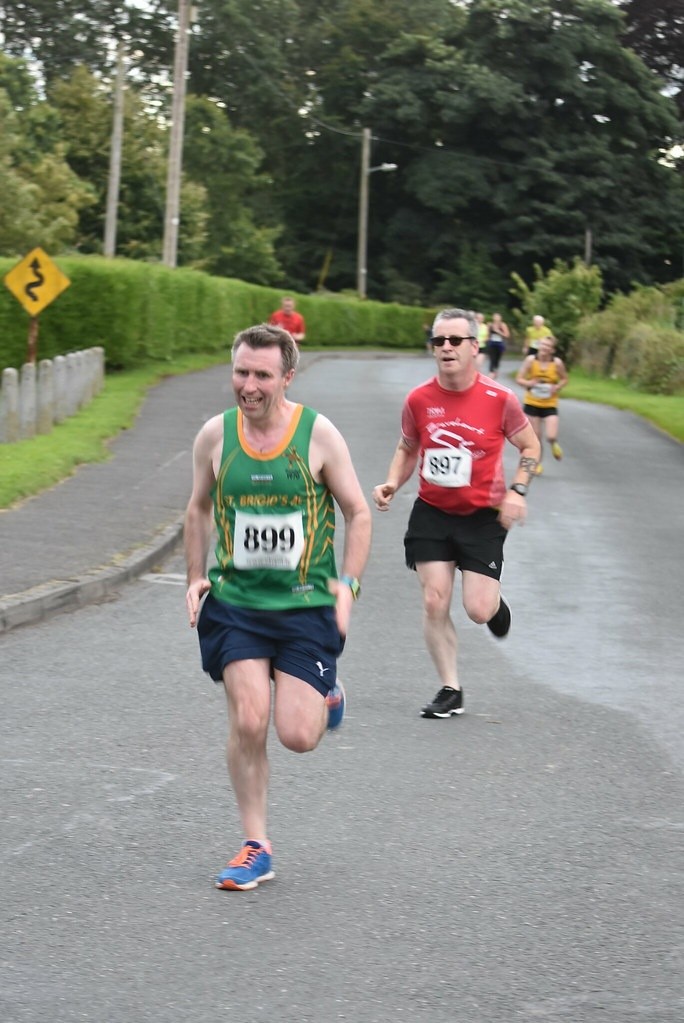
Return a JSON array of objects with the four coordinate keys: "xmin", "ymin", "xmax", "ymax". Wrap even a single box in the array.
[
  {"xmin": 486, "ymin": 590, "xmax": 513, "ymax": 638},
  {"xmin": 326, "ymin": 678, "xmax": 346, "ymax": 730},
  {"xmin": 419, "ymin": 685, "xmax": 465, "ymax": 718},
  {"xmin": 216, "ymin": 839, "xmax": 275, "ymax": 891}
]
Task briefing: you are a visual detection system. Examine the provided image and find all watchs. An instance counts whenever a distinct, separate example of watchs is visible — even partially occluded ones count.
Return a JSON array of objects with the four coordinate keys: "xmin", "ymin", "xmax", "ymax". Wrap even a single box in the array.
[{"xmin": 511, "ymin": 482, "xmax": 529, "ymax": 497}]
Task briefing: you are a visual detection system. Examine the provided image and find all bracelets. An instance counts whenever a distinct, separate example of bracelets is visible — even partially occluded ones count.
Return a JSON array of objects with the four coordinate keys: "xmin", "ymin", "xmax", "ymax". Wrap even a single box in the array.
[{"xmin": 341, "ymin": 576, "xmax": 363, "ymax": 599}]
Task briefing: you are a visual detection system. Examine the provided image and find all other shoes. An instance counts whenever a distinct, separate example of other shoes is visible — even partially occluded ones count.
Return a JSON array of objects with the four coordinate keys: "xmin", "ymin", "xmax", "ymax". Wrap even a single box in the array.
[
  {"xmin": 534, "ymin": 464, "xmax": 543, "ymax": 476},
  {"xmin": 549, "ymin": 443, "xmax": 563, "ymax": 460},
  {"xmin": 490, "ymin": 371, "xmax": 497, "ymax": 379}
]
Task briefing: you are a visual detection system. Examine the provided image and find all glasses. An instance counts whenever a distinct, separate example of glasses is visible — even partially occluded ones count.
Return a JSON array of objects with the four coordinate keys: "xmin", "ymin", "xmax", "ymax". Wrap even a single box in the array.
[{"xmin": 430, "ymin": 336, "xmax": 475, "ymax": 346}]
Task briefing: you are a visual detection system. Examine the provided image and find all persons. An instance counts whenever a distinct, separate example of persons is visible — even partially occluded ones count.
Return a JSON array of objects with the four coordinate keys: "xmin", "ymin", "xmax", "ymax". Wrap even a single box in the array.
[
  {"xmin": 184, "ymin": 325, "xmax": 371, "ymax": 888},
  {"xmin": 522, "ymin": 313, "xmax": 556, "ymax": 355},
  {"xmin": 270, "ymin": 298, "xmax": 307, "ymax": 343},
  {"xmin": 486, "ymin": 311, "xmax": 510, "ymax": 381},
  {"xmin": 473, "ymin": 312, "xmax": 488, "ymax": 369},
  {"xmin": 375, "ymin": 308, "xmax": 542, "ymax": 722},
  {"xmin": 421, "ymin": 322, "xmax": 433, "ymax": 354},
  {"xmin": 516, "ymin": 336, "xmax": 568, "ymax": 478}
]
